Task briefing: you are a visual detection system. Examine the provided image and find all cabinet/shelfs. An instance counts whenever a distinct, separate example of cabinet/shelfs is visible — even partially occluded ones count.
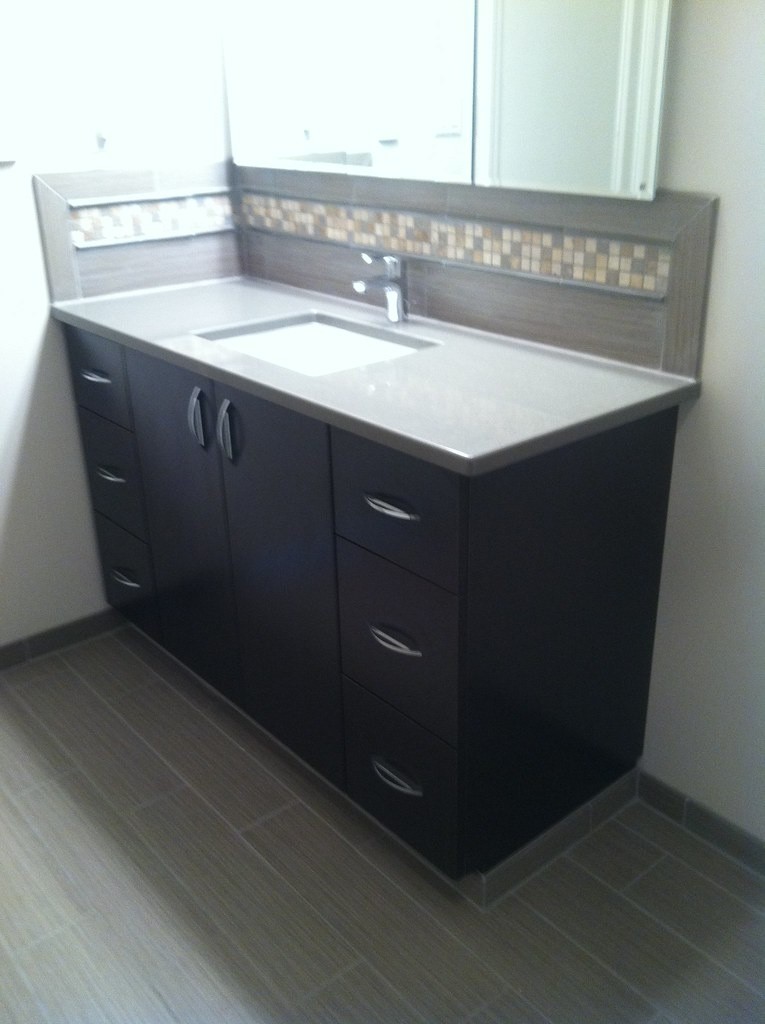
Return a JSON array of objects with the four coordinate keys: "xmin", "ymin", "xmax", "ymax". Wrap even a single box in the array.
[
  {"xmin": 328, "ymin": 413, "xmax": 681, "ymax": 904},
  {"xmin": 60, "ymin": 321, "xmax": 157, "ymax": 643},
  {"xmin": 124, "ymin": 341, "xmax": 348, "ymax": 811}
]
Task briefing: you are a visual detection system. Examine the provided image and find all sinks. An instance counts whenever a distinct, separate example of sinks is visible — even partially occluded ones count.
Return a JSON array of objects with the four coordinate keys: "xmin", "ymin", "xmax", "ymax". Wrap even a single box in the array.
[{"xmin": 194, "ymin": 311, "xmax": 439, "ymax": 377}]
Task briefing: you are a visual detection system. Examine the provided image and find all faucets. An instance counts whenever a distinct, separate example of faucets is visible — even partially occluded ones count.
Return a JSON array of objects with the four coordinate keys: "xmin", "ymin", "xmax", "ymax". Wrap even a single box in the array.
[{"xmin": 353, "ymin": 250, "xmax": 410, "ymax": 323}]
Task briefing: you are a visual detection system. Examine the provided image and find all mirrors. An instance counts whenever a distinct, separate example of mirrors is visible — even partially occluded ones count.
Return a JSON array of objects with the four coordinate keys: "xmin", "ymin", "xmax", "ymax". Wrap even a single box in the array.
[{"xmin": 225, "ymin": 0, "xmax": 673, "ymax": 204}]
